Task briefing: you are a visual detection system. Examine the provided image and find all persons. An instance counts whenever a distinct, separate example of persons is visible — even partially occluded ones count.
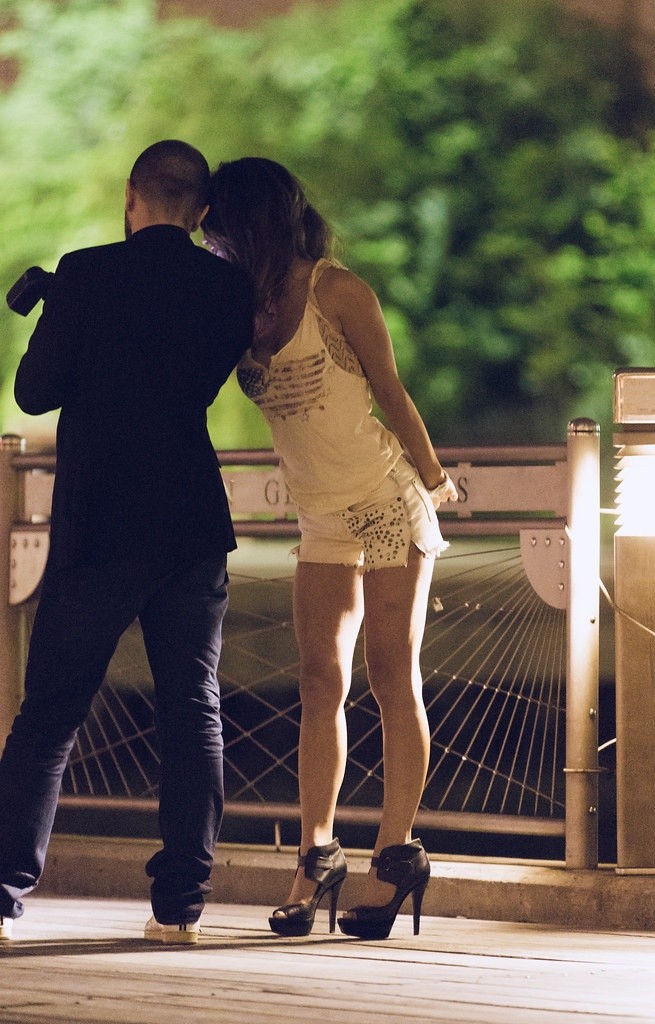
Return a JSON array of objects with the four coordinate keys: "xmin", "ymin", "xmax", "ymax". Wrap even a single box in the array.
[
  {"xmin": 201, "ymin": 156, "xmax": 458, "ymax": 941},
  {"xmin": 0, "ymin": 139, "xmax": 254, "ymax": 945}
]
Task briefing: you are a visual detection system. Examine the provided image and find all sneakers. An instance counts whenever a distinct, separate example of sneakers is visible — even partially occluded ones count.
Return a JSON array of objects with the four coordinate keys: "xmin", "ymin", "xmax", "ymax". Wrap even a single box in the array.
[
  {"xmin": 0, "ymin": 916, "xmax": 13, "ymax": 940},
  {"xmin": 144, "ymin": 915, "xmax": 200, "ymax": 945}
]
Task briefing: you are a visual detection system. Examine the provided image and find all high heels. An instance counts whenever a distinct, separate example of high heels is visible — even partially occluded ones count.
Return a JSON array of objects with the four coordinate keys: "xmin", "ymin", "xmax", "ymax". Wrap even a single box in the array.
[
  {"xmin": 268, "ymin": 837, "xmax": 347, "ymax": 936},
  {"xmin": 337, "ymin": 838, "xmax": 430, "ymax": 941}
]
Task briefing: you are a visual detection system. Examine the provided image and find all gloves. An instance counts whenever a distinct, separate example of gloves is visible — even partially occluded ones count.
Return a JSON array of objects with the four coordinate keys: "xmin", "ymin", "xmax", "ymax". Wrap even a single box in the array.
[{"xmin": 426, "ymin": 471, "xmax": 458, "ymax": 511}]
